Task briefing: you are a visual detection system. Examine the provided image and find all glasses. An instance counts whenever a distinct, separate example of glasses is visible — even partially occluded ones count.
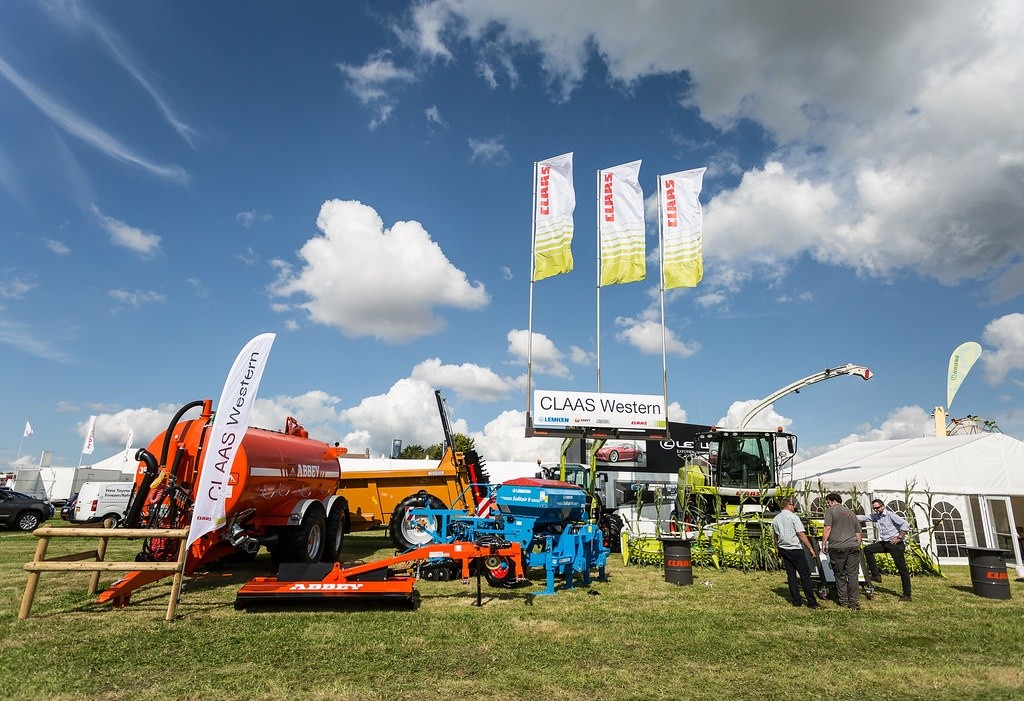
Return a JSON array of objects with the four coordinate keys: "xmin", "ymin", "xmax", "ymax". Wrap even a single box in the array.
[
  {"xmin": 872, "ymin": 505, "xmax": 883, "ymax": 509},
  {"xmin": 789, "ymin": 503, "xmax": 794, "ymax": 507}
]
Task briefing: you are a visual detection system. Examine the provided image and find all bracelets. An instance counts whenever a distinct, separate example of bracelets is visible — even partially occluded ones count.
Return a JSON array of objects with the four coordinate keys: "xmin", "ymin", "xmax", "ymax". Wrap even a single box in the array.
[{"xmin": 897, "ymin": 536, "xmax": 902, "ymax": 539}]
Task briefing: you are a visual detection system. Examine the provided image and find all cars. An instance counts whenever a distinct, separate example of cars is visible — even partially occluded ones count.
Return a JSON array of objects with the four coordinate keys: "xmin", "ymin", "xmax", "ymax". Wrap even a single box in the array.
[
  {"xmin": 61, "ymin": 492, "xmax": 79, "ymax": 521},
  {"xmin": 0, "ymin": 489, "xmax": 50, "ymax": 532},
  {"xmin": 5, "ymin": 491, "xmax": 55, "ymax": 519},
  {"xmin": 596, "ymin": 442, "xmax": 643, "ymax": 463}
]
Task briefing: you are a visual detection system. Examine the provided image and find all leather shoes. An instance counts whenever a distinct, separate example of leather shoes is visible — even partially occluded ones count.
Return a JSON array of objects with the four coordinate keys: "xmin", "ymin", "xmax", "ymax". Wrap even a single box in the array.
[
  {"xmin": 897, "ymin": 595, "xmax": 913, "ymax": 602},
  {"xmin": 816, "ymin": 604, "xmax": 827, "ymax": 609},
  {"xmin": 870, "ymin": 576, "xmax": 882, "ymax": 583}
]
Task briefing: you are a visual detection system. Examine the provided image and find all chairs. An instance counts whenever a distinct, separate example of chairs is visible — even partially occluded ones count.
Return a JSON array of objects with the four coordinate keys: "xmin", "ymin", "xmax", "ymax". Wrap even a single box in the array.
[{"xmin": 720, "ymin": 439, "xmax": 741, "ymax": 462}]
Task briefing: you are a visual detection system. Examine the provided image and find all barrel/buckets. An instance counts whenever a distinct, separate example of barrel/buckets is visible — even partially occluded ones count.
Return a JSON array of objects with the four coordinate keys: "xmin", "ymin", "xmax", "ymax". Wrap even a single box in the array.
[
  {"xmin": 664, "ymin": 541, "xmax": 693, "ymax": 586},
  {"xmin": 968, "ymin": 549, "xmax": 1011, "ymax": 599}
]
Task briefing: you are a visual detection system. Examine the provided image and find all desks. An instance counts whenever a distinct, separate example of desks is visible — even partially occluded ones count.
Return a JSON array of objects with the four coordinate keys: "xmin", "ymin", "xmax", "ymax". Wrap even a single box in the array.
[{"xmin": 998, "ymin": 532, "xmax": 1024, "ymax": 559}]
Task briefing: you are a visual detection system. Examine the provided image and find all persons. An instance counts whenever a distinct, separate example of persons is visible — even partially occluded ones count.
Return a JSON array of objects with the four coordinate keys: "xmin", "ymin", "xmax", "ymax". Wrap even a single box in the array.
[
  {"xmin": 822, "ymin": 493, "xmax": 862, "ymax": 611},
  {"xmin": 771, "ymin": 498, "xmax": 821, "ymax": 608},
  {"xmin": 856, "ymin": 499, "xmax": 914, "ymax": 602}
]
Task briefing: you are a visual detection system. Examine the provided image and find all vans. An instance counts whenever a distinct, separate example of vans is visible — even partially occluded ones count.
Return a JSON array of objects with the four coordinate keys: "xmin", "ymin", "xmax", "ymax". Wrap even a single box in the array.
[{"xmin": 70, "ymin": 481, "xmax": 135, "ymax": 529}]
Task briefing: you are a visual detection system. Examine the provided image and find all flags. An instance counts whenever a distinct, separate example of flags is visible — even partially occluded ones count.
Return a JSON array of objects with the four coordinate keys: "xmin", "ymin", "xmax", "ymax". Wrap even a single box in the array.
[
  {"xmin": 83, "ymin": 415, "xmax": 95, "ymax": 454},
  {"xmin": 124, "ymin": 430, "xmax": 133, "ymax": 461},
  {"xmin": 661, "ymin": 167, "xmax": 707, "ymax": 289},
  {"xmin": 600, "ymin": 159, "xmax": 646, "ymax": 286},
  {"xmin": 532, "ymin": 152, "xmax": 576, "ymax": 281},
  {"xmin": 190, "ymin": 334, "xmax": 274, "ymax": 549},
  {"xmin": 23, "ymin": 421, "xmax": 33, "ymax": 438}
]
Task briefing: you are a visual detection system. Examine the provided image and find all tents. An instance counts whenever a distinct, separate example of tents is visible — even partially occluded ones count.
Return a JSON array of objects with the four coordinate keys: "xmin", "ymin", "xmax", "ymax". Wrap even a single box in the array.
[{"xmin": 780, "ymin": 433, "xmax": 1024, "ymax": 566}]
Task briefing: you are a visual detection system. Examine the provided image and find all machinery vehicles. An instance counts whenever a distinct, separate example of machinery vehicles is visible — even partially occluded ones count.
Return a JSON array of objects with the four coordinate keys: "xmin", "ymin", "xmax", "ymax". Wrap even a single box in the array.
[{"xmin": 621, "ymin": 425, "xmax": 863, "ymax": 569}]
